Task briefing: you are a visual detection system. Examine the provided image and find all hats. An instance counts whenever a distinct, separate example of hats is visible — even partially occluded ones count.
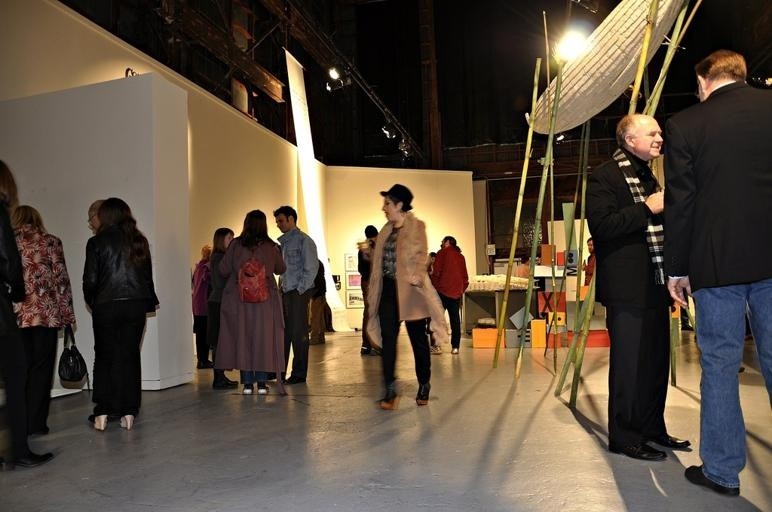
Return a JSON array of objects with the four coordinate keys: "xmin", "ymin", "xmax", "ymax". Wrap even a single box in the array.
[
  {"xmin": 380, "ymin": 184, "xmax": 414, "ymax": 211},
  {"xmin": 365, "ymin": 225, "xmax": 379, "ymax": 238}
]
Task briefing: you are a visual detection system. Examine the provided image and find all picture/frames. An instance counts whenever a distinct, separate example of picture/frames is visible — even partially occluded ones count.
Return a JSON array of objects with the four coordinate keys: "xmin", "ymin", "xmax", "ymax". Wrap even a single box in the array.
[{"xmin": 346, "ymin": 254, "xmax": 364, "ymax": 309}]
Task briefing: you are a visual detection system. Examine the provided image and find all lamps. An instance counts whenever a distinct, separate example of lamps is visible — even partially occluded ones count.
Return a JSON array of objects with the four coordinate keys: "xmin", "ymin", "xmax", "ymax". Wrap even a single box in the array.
[
  {"xmin": 328, "ymin": 64, "xmax": 343, "ymax": 79},
  {"xmin": 325, "ymin": 77, "xmax": 352, "ymax": 93},
  {"xmin": 381, "ymin": 124, "xmax": 396, "ymax": 139}
]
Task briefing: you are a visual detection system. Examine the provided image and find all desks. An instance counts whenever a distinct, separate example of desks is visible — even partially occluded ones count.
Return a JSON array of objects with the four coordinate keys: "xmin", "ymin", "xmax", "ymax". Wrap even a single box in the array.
[{"xmin": 461, "ymin": 289, "xmax": 537, "ymax": 338}]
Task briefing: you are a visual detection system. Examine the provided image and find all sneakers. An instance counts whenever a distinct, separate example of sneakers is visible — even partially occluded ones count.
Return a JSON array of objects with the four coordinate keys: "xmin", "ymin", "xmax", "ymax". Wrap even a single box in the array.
[
  {"xmin": 430, "ymin": 345, "xmax": 442, "ymax": 355},
  {"xmin": 450, "ymin": 347, "xmax": 459, "ymax": 354}
]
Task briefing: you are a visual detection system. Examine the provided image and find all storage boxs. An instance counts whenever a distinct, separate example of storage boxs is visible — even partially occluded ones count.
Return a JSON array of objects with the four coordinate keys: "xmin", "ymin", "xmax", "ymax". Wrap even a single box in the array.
[
  {"xmin": 557, "ymin": 252, "xmax": 565, "ymax": 265},
  {"xmin": 539, "ymin": 244, "xmax": 556, "ymax": 266},
  {"xmin": 472, "ymin": 290, "xmax": 610, "ymax": 349}
]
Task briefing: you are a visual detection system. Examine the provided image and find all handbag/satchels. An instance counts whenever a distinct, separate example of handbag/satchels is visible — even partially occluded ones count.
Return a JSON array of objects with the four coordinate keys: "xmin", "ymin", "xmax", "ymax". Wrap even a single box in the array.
[{"xmin": 58, "ymin": 325, "xmax": 90, "ymax": 389}]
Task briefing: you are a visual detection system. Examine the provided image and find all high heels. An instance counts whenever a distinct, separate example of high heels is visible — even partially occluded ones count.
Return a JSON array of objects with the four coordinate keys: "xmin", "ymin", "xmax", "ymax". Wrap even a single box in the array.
[
  {"xmin": 415, "ymin": 382, "xmax": 431, "ymax": 405},
  {"xmin": 120, "ymin": 413, "xmax": 134, "ymax": 430},
  {"xmin": 94, "ymin": 415, "xmax": 108, "ymax": 431},
  {"xmin": 375, "ymin": 391, "xmax": 400, "ymax": 410}
]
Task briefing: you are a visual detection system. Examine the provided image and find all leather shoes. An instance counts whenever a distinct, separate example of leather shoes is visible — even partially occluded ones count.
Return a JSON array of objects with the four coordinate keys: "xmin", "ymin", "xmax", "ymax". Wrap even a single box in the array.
[
  {"xmin": 360, "ymin": 348, "xmax": 377, "ymax": 355},
  {"xmin": 650, "ymin": 434, "xmax": 691, "ymax": 448},
  {"xmin": 608, "ymin": 443, "xmax": 667, "ymax": 461},
  {"xmin": 0, "ymin": 449, "xmax": 53, "ymax": 471},
  {"xmin": 685, "ymin": 465, "xmax": 740, "ymax": 497},
  {"xmin": 197, "ymin": 359, "xmax": 213, "ymax": 369},
  {"xmin": 212, "ymin": 369, "xmax": 307, "ymax": 395}
]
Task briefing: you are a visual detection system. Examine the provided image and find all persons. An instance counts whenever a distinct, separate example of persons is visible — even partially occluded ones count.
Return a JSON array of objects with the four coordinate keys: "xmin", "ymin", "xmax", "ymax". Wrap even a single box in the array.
[
  {"xmin": 1, "ymin": 161, "xmax": 76, "ymax": 471},
  {"xmin": 659, "ymin": 50, "xmax": 772, "ymax": 496},
  {"xmin": 429, "ymin": 235, "xmax": 469, "ymax": 354},
  {"xmin": 585, "ymin": 112, "xmax": 690, "ymax": 462},
  {"xmin": 83, "ymin": 198, "xmax": 160, "ymax": 431},
  {"xmin": 428, "ymin": 252, "xmax": 437, "ymax": 275},
  {"xmin": 191, "ymin": 206, "xmax": 335, "ymax": 395},
  {"xmin": 365, "ymin": 183, "xmax": 451, "ymax": 410},
  {"xmin": 579, "ymin": 236, "xmax": 596, "ymax": 285},
  {"xmin": 356, "ymin": 225, "xmax": 383, "ymax": 357}
]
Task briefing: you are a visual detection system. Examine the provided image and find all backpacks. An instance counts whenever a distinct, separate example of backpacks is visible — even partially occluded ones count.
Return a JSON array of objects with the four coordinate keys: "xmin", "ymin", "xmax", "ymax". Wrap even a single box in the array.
[{"xmin": 237, "ymin": 260, "xmax": 270, "ymax": 303}]
